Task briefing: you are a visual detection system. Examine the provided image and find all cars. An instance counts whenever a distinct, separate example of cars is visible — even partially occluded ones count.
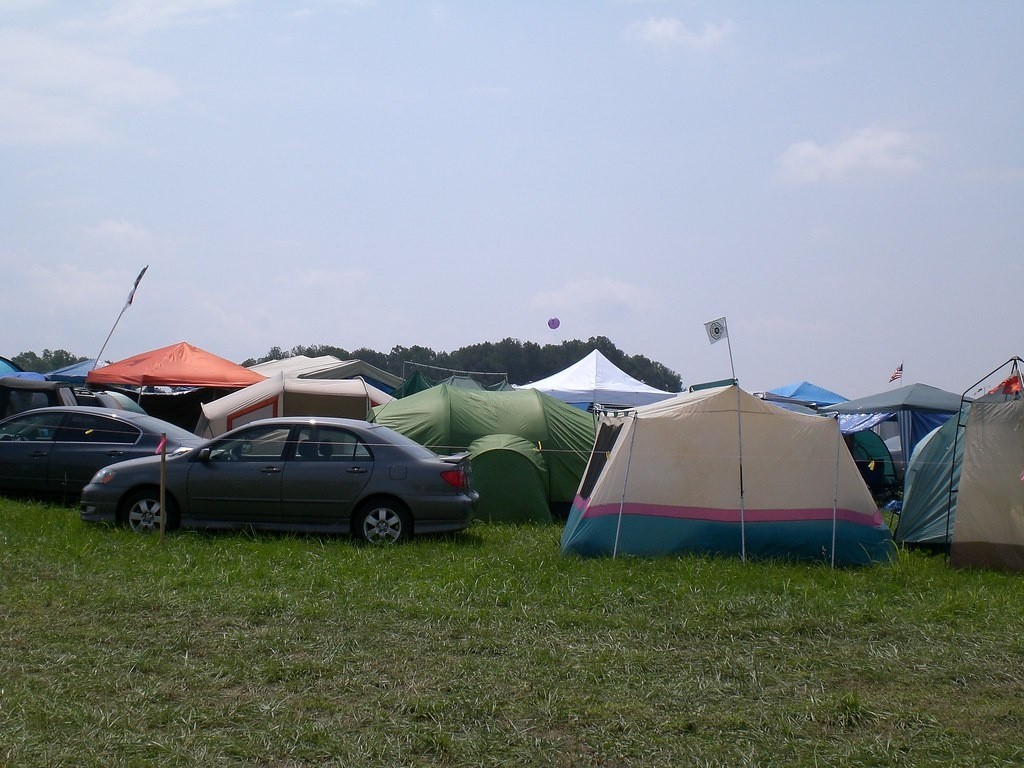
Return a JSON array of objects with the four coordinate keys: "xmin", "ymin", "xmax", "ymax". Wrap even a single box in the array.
[
  {"xmin": 0, "ymin": 376, "xmax": 211, "ymax": 495},
  {"xmin": 79, "ymin": 416, "xmax": 480, "ymax": 543}
]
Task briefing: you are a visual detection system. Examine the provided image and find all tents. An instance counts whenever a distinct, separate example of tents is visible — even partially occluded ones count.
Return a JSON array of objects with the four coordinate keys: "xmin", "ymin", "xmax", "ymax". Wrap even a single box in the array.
[{"xmin": 0, "ymin": 342, "xmax": 1023, "ymax": 575}]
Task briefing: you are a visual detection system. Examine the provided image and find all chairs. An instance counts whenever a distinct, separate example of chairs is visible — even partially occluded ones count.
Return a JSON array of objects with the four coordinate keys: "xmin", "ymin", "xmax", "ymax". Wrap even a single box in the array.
[
  {"xmin": 319, "ymin": 439, "xmax": 334, "ymax": 462},
  {"xmin": 298, "ymin": 439, "xmax": 318, "ymax": 461}
]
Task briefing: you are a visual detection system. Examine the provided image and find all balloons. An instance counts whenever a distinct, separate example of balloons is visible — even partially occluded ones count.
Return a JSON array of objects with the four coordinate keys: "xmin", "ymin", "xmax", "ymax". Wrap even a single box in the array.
[{"xmin": 548, "ymin": 317, "xmax": 560, "ymax": 329}]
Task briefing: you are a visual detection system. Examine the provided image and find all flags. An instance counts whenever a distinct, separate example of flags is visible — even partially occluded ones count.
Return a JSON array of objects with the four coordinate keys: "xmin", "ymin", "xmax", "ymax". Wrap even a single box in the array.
[
  {"xmin": 704, "ymin": 318, "xmax": 728, "ymax": 343},
  {"xmin": 887, "ymin": 363, "xmax": 902, "ymax": 383}
]
